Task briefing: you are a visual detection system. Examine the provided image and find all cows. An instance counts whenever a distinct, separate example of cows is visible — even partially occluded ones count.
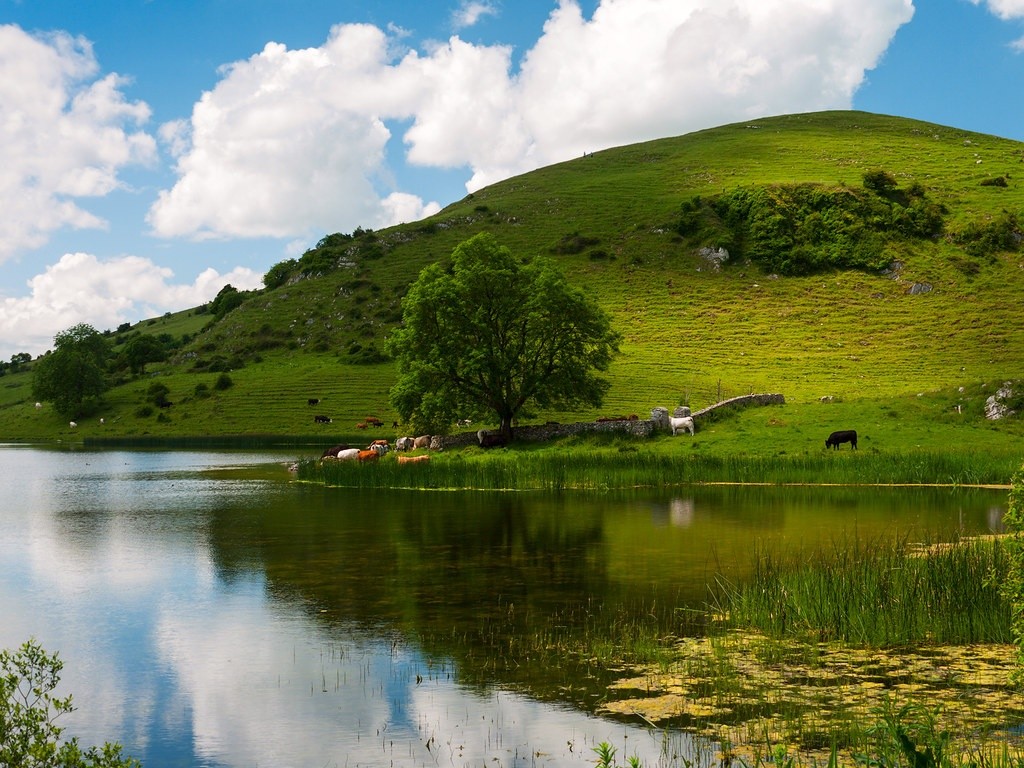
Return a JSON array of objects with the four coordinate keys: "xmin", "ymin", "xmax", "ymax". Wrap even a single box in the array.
[
  {"xmin": 321, "ymin": 434, "xmax": 443, "ymax": 466},
  {"xmin": 392, "ymin": 422, "xmax": 399, "ymax": 428},
  {"xmin": 308, "ymin": 399, "xmax": 318, "ymax": 406},
  {"xmin": 825, "ymin": 430, "xmax": 857, "ymax": 451},
  {"xmin": 455, "ymin": 419, "xmax": 472, "ymax": 428},
  {"xmin": 314, "ymin": 415, "xmax": 330, "ymax": 423},
  {"xmin": 365, "ymin": 417, "xmax": 384, "ymax": 428},
  {"xmin": 356, "ymin": 423, "xmax": 368, "ymax": 429}
]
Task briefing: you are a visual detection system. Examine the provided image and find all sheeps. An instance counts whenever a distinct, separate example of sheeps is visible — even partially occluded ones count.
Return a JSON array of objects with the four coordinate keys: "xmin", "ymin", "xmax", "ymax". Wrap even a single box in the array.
[
  {"xmin": 35, "ymin": 402, "xmax": 42, "ymax": 409},
  {"xmin": 100, "ymin": 418, "xmax": 104, "ymax": 423},
  {"xmin": 669, "ymin": 417, "xmax": 694, "ymax": 436},
  {"xmin": 69, "ymin": 422, "xmax": 77, "ymax": 428},
  {"xmin": 477, "ymin": 429, "xmax": 487, "ymax": 444}
]
